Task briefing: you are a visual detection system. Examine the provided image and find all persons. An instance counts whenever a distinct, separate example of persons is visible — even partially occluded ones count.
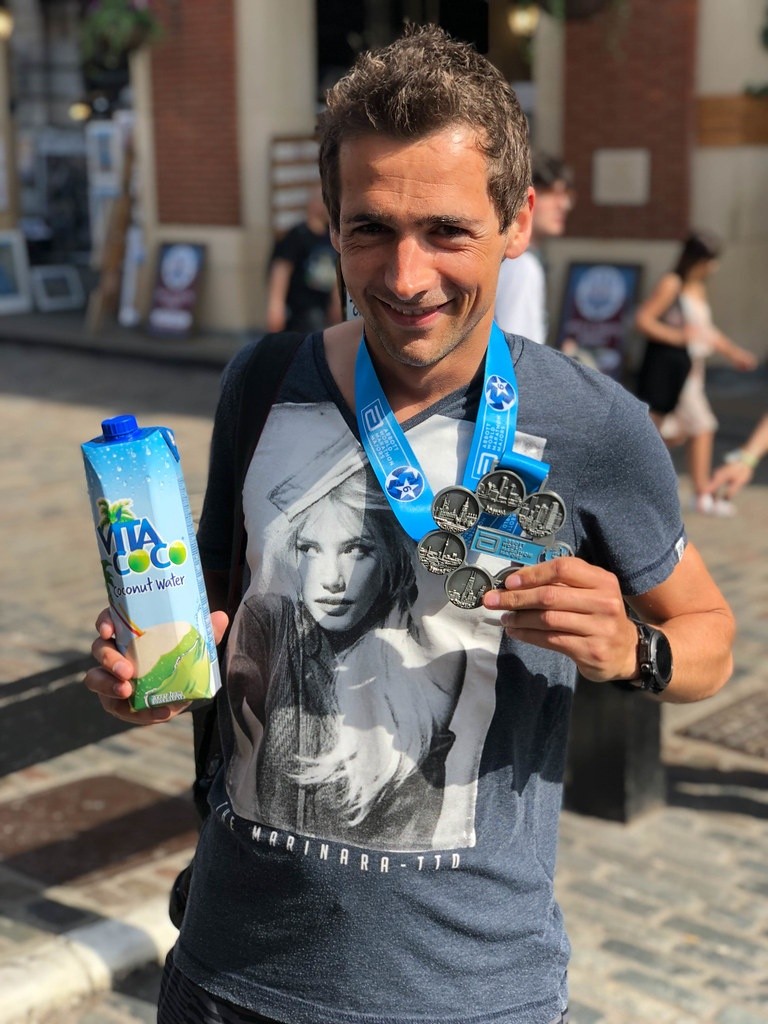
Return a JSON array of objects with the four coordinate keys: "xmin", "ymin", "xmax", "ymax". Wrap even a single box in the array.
[
  {"xmin": 699, "ymin": 415, "xmax": 768, "ymax": 499},
  {"xmin": 262, "ymin": 184, "xmax": 343, "ymax": 332},
  {"xmin": 635, "ymin": 229, "xmax": 756, "ymax": 516},
  {"xmin": 88, "ymin": 21, "xmax": 738, "ymax": 1024},
  {"xmin": 228, "ymin": 466, "xmax": 467, "ymax": 852},
  {"xmin": 495, "ymin": 153, "xmax": 574, "ymax": 346}
]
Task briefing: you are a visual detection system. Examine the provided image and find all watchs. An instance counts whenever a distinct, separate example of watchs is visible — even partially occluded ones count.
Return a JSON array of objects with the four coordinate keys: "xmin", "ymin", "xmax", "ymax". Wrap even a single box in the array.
[{"xmin": 613, "ymin": 615, "xmax": 674, "ymax": 696}]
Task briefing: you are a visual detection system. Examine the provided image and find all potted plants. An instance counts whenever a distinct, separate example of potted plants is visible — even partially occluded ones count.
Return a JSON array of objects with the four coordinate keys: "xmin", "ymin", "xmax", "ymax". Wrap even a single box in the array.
[{"xmin": 72, "ymin": 4, "xmax": 157, "ymax": 88}]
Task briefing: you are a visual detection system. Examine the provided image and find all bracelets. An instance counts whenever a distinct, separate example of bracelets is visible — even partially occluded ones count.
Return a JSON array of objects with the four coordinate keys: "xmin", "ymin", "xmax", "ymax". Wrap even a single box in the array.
[{"xmin": 722, "ymin": 449, "xmax": 761, "ymax": 469}]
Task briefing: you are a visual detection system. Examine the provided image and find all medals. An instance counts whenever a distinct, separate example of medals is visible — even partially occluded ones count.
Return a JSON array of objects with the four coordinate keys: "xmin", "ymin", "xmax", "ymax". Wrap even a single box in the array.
[{"xmin": 416, "ymin": 470, "xmax": 576, "ymax": 609}]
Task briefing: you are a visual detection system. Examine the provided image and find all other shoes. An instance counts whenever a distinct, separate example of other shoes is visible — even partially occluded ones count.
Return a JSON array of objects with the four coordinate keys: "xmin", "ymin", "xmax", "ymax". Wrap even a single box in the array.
[{"xmin": 689, "ymin": 493, "xmax": 733, "ymax": 517}]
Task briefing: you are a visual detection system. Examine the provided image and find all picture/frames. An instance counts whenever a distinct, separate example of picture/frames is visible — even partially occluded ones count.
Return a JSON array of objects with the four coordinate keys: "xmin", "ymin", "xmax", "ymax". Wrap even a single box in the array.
[
  {"xmin": 144, "ymin": 242, "xmax": 208, "ymax": 341},
  {"xmin": 0, "ymin": 228, "xmax": 33, "ymax": 315},
  {"xmin": 30, "ymin": 264, "xmax": 84, "ymax": 312},
  {"xmin": 552, "ymin": 259, "xmax": 643, "ymax": 379}
]
